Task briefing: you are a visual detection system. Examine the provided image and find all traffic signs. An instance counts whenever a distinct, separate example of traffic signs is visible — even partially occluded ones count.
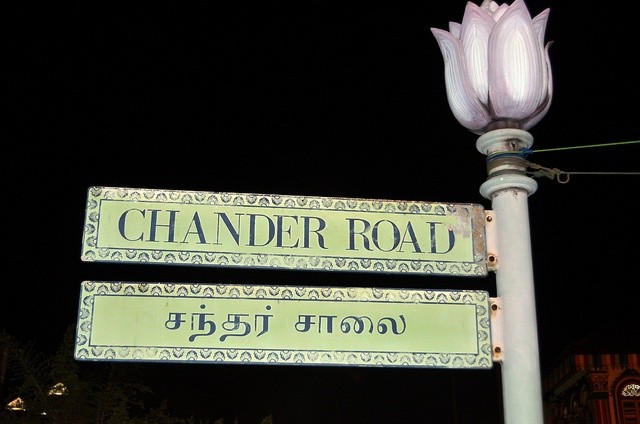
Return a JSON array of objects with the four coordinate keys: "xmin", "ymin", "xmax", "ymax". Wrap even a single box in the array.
[
  {"xmin": 80, "ymin": 185, "xmax": 490, "ymax": 279},
  {"xmin": 73, "ymin": 279, "xmax": 495, "ymax": 372}
]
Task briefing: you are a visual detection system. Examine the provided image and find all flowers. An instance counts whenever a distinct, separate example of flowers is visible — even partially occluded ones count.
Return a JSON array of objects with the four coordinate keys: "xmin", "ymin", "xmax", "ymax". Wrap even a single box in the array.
[{"xmin": 430, "ymin": 0, "xmax": 556, "ymax": 135}]
